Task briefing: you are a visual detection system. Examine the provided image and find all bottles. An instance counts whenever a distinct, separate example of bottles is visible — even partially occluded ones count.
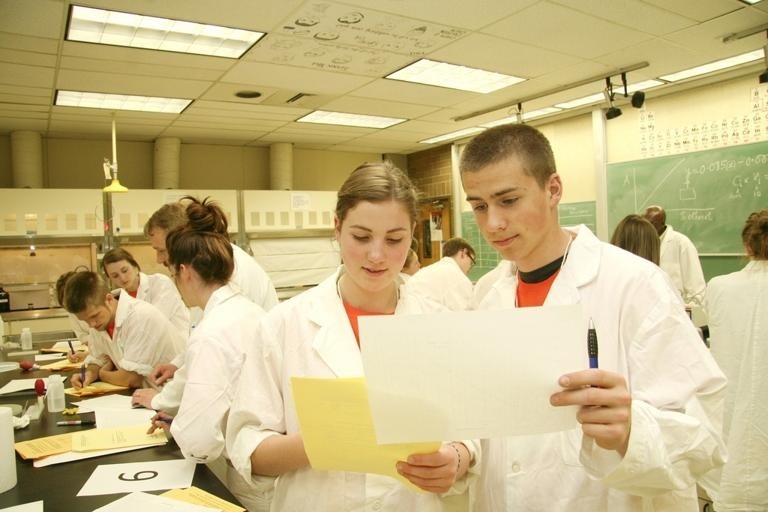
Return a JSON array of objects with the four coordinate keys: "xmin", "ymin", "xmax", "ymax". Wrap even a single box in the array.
[
  {"xmin": 0, "ymin": 407, "xmax": 17, "ymax": 494},
  {"xmin": 46, "ymin": 375, "xmax": 65, "ymax": 412},
  {"xmin": 20, "ymin": 328, "xmax": 32, "ymax": 350}
]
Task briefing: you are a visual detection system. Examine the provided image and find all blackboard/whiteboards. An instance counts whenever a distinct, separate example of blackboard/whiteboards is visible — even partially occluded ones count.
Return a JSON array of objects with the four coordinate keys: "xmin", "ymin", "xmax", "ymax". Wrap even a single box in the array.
[
  {"xmin": 462, "ymin": 201, "xmax": 598, "ymax": 284},
  {"xmin": 605, "ymin": 143, "xmax": 767, "ymax": 257}
]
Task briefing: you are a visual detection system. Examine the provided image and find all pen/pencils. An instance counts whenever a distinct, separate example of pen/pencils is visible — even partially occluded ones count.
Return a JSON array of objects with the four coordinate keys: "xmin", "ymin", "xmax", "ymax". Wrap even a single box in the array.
[
  {"xmin": 588, "ymin": 318, "xmax": 598, "ymax": 388},
  {"xmin": 82, "ymin": 364, "xmax": 85, "ymax": 387},
  {"xmin": 56, "ymin": 421, "xmax": 96, "ymax": 425},
  {"xmin": 150, "ymin": 417, "xmax": 173, "ymax": 422},
  {"xmin": 68, "ymin": 341, "xmax": 74, "ymax": 354}
]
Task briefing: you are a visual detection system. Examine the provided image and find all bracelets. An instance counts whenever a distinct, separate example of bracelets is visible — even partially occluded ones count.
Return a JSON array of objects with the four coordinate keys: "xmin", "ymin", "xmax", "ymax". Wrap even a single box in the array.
[{"xmin": 446, "ymin": 443, "xmax": 462, "ymax": 473}]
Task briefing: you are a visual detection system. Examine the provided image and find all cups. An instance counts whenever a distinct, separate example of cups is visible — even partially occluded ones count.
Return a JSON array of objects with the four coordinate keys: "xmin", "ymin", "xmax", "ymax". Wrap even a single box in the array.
[{"xmin": 28, "ymin": 303, "xmax": 33, "ymax": 310}]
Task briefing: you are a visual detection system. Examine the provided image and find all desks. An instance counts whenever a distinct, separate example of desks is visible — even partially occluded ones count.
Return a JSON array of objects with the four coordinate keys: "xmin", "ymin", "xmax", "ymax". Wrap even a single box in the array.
[{"xmin": 0, "ymin": 330, "xmax": 251, "ymax": 512}]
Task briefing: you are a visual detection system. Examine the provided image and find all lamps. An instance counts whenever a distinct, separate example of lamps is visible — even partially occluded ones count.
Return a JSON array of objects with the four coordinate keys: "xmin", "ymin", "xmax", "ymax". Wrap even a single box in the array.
[
  {"xmin": 604, "ymin": 73, "xmax": 645, "ymax": 119},
  {"xmin": 103, "ymin": 111, "xmax": 128, "ymax": 193}
]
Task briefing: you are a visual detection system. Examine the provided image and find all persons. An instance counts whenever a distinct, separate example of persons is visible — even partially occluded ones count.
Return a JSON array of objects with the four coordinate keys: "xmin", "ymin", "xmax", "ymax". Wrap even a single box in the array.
[
  {"xmin": 395, "ymin": 236, "xmax": 476, "ymax": 314},
  {"xmin": 400, "ymin": 248, "xmax": 421, "ymax": 282},
  {"xmin": 221, "ymin": 159, "xmax": 485, "ymax": 512},
  {"xmin": 51, "ymin": 195, "xmax": 280, "ymax": 509},
  {"xmin": 642, "ymin": 205, "xmax": 707, "ymax": 305},
  {"xmin": 682, "ymin": 209, "xmax": 768, "ymax": 511},
  {"xmin": 456, "ymin": 122, "xmax": 730, "ymax": 511},
  {"xmin": 609, "ymin": 214, "xmax": 660, "ymax": 267}
]
